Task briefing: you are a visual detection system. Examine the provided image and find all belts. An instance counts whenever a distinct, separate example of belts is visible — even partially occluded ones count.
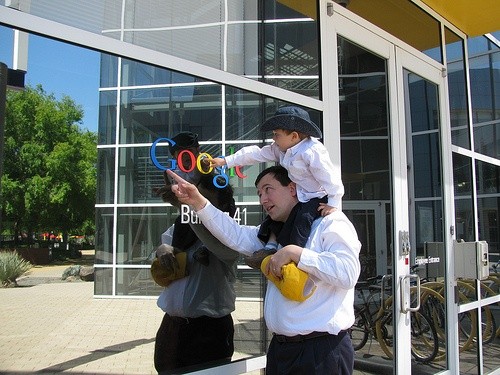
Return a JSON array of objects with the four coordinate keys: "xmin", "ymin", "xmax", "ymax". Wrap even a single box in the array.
[
  {"xmin": 275, "ymin": 330, "xmax": 344, "ymax": 343},
  {"xmin": 164, "ymin": 312, "xmax": 232, "ymax": 327}
]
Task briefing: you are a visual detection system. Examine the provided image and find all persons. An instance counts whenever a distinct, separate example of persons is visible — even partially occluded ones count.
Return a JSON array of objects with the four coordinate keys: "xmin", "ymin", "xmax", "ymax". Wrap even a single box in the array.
[
  {"xmin": 152, "ymin": 185, "xmax": 237, "ymax": 375},
  {"xmin": 203, "ymin": 107, "xmax": 345, "ymax": 270},
  {"xmin": 165, "ymin": 164, "xmax": 362, "ymax": 375},
  {"xmin": 169, "ymin": 131, "xmax": 236, "ymax": 266}
]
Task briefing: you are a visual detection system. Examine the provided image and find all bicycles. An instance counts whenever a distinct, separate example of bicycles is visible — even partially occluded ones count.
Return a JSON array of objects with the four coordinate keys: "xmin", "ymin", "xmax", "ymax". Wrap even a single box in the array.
[{"xmin": 346, "ymin": 263, "xmax": 500, "ymax": 364}]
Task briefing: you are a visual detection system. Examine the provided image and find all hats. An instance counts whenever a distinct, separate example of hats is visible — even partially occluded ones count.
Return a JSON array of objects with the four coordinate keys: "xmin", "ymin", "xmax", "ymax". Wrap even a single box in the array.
[
  {"xmin": 152, "ymin": 251, "xmax": 187, "ymax": 286},
  {"xmin": 261, "ymin": 255, "xmax": 317, "ymax": 301},
  {"xmin": 260, "ymin": 107, "xmax": 322, "ymax": 138},
  {"xmin": 168, "ymin": 132, "xmax": 201, "ymax": 154}
]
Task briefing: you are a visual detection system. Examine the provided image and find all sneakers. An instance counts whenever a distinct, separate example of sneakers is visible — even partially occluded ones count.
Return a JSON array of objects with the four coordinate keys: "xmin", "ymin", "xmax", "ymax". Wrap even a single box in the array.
[
  {"xmin": 245, "ymin": 248, "xmax": 276, "ymax": 268},
  {"xmin": 192, "ymin": 247, "xmax": 209, "ymax": 267}
]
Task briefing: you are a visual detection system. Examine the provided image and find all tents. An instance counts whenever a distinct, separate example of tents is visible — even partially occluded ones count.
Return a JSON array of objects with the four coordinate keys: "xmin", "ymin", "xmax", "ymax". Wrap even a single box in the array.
[
  {"xmin": 40, "ymin": 231, "xmax": 57, "ymax": 244},
  {"xmin": 56, "ymin": 233, "xmax": 84, "ymax": 245}
]
